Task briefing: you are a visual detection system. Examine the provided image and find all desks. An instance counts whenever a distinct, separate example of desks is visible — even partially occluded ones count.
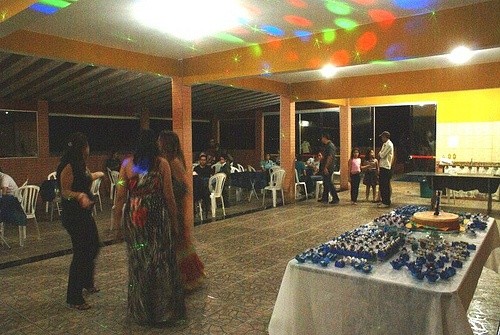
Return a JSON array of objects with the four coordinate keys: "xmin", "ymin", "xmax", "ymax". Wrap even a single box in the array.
[
  {"xmin": 225, "ymin": 172, "xmax": 268, "ymax": 203},
  {"xmin": 40, "ymin": 179, "xmax": 61, "ymax": 220},
  {"xmin": 193, "ymin": 175, "xmax": 210, "ymax": 220},
  {"xmin": 269, "ymin": 206, "xmax": 500, "ymax": 335},
  {"xmin": 426, "ymin": 173, "xmax": 500, "ymax": 213},
  {"xmin": 0, "ymin": 194, "xmax": 27, "ymax": 227}
]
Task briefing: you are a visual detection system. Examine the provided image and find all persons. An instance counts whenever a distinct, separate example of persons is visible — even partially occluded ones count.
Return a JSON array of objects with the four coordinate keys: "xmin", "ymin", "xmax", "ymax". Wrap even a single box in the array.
[
  {"xmin": 308, "ymin": 131, "xmax": 340, "ymax": 204},
  {"xmin": 192, "ymin": 152, "xmax": 232, "ymax": 218},
  {"xmin": 378, "ymin": 130, "xmax": 394, "ymax": 208},
  {"xmin": 0, "ymin": 171, "xmax": 23, "ymax": 207},
  {"xmin": 361, "ymin": 149, "xmax": 379, "ymax": 203},
  {"xmin": 57, "ymin": 133, "xmax": 105, "ymax": 310},
  {"xmin": 110, "ymin": 132, "xmax": 203, "ymax": 326},
  {"xmin": 347, "ymin": 148, "xmax": 370, "ymax": 203},
  {"xmin": 258, "ymin": 152, "xmax": 276, "ymax": 172},
  {"xmin": 293, "ymin": 154, "xmax": 313, "ymax": 194}
]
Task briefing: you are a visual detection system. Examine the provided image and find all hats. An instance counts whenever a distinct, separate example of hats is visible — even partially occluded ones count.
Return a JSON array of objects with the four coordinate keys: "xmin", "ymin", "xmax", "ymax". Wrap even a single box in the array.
[{"xmin": 379, "ymin": 131, "xmax": 390, "ymax": 138}]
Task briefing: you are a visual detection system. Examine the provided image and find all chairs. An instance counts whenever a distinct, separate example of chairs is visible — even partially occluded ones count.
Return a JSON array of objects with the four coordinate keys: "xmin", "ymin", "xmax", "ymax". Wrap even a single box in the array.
[
  {"xmin": 295, "ymin": 169, "xmax": 307, "ymax": 200},
  {"xmin": 332, "ymin": 170, "xmax": 340, "ymax": 182},
  {"xmin": 316, "ymin": 181, "xmax": 329, "ymax": 199},
  {"xmin": 1, "ymin": 172, "xmax": 62, "ymax": 247},
  {"xmin": 90, "ymin": 177, "xmax": 102, "ymax": 216},
  {"xmin": 193, "ymin": 163, "xmax": 286, "ymax": 221},
  {"xmin": 106, "ymin": 167, "xmax": 126, "ymax": 231}
]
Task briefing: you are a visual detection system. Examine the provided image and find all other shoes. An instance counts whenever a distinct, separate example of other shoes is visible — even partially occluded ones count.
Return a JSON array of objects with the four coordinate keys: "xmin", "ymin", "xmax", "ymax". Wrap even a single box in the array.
[
  {"xmin": 81, "ymin": 285, "xmax": 100, "ymax": 293},
  {"xmin": 70, "ymin": 302, "xmax": 92, "ymax": 311},
  {"xmin": 378, "ymin": 203, "xmax": 390, "ymax": 208},
  {"xmin": 317, "ymin": 198, "xmax": 328, "ymax": 203},
  {"xmin": 329, "ymin": 198, "xmax": 340, "ymax": 204}
]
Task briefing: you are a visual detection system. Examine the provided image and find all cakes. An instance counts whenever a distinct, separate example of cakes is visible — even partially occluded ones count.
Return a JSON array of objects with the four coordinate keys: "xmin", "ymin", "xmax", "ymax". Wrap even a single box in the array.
[{"xmin": 411, "ymin": 210, "xmax": 460, "ymax": 232}]
